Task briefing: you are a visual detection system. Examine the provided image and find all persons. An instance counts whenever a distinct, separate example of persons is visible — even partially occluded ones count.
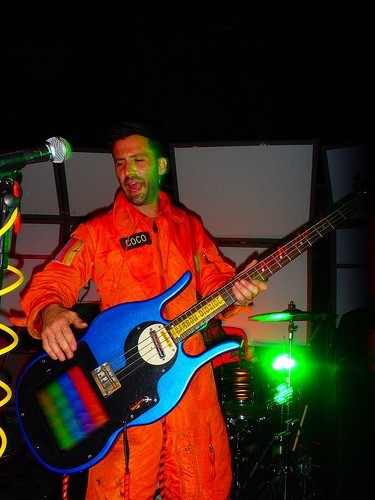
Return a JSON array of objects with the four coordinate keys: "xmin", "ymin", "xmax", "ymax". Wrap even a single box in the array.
[
  {"xmin": 19, "ymin": 125, "xmax": 268, "ymax": 500},
  {"xmin": 202, "ymin": 317, "xmax": 227, "ymax": 349}
]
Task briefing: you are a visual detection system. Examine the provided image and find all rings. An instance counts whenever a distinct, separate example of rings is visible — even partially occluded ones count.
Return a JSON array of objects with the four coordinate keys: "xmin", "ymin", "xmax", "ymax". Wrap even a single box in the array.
[{"xmin": 246, "ymin": 295, "xmax": 253, "ymax": 301}]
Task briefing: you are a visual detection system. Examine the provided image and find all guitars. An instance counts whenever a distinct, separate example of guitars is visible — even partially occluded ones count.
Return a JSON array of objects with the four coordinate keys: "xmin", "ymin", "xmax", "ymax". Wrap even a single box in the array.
[{"xmin": 14, "ymin": 184, "xmax": 375, "ymax": 476}]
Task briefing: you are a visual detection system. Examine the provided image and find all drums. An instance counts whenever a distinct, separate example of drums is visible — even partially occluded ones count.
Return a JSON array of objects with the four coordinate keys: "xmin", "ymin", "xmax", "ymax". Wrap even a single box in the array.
[{"xmin": 214, "ymin": 360, "xmax": 267, "ymax": 408}]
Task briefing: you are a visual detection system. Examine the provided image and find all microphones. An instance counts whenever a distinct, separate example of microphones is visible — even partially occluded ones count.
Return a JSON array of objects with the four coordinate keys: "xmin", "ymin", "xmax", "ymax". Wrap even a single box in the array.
[{"xmin": 0, "ymin": 136, "xmax": 73, "ymax": 170}]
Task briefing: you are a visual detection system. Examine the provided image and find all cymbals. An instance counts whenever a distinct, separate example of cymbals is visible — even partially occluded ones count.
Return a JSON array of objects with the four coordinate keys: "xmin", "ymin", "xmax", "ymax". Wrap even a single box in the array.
[{"xmin": 247, "ymin": 310, "xmax": 341, "ymax": 322}]
